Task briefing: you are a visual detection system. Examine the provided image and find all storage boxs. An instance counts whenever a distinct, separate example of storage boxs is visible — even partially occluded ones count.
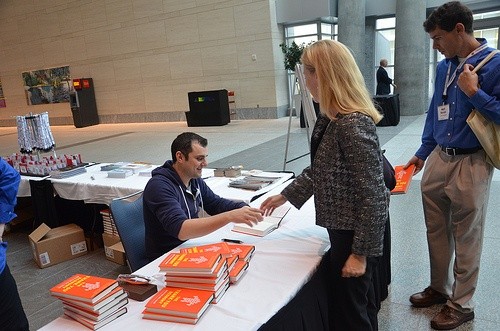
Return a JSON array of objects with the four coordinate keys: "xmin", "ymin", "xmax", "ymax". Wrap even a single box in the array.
[
  {"xmin": 107, "ymin": 240, "xmax": 133, "ymax": 274},
  {"xmin": 102, "ymin": 232, "xmax": 128, "ymax": 266},
  {"xmin": 29, "ymin": 223, "xmax": 88, "ymax": 269}
]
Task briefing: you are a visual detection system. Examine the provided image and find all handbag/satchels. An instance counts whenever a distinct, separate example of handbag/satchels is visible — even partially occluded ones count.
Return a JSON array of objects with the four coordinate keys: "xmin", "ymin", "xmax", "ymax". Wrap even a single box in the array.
[
  {"xmin": 466, "ymin": 50, "xmax": 500, "ymax": 171},
  {"xmin": 382, "ymin": 155, "xmax": 397, "ymax": 191}
]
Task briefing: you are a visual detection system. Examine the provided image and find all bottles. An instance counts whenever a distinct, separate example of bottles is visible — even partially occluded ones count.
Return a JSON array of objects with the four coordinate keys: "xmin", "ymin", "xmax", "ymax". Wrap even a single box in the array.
[{"xmin": 7, "ymin": 152, "xmax": 82, "ymax": 175}]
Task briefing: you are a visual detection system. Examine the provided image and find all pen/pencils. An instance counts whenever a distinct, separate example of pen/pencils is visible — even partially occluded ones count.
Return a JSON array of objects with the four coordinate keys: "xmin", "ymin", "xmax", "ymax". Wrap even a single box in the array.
[{"xmin": 221, "ymin": 238, "xmax": 244, "ymax": 244}]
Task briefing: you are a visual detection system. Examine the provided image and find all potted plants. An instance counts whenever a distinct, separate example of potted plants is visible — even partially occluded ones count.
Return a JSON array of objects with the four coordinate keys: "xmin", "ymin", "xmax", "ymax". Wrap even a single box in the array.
[{"xmin": 279, "ymin": 40, "xmax": 320, "ymax": 128}]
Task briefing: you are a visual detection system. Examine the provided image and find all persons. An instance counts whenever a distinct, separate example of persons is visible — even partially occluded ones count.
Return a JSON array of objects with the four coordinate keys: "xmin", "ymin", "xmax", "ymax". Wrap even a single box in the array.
[
  {"xmin": 143, "ymin": 133, "xmax": 263, "ymax": 260},
  {"xmin": 403, "ymin": 1, "xmax": 500, "ymax": 330},
  {"xmin": 261, "ymin": 41, "xmax": 390, "ymax": 330},
  {"xmin": 0, "ymin": 155, "xmax": 29, "ymax": 330},
  {"xmin": 376, "ymin": 58, "xmax": 397, "ymax": 95}
]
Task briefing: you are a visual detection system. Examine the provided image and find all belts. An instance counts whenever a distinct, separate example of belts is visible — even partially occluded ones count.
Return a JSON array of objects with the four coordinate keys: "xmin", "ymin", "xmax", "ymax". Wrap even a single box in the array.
[{"xmin": 439, "ymin": 144, "xmax": 484, "ymax": 156}]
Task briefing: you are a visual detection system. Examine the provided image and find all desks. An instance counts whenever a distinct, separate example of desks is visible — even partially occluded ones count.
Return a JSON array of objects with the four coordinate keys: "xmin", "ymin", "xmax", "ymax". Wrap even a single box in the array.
[
  {"xmin": 373, "ymin": 92, "xmax": 400, "ymax": 127},
  {"xmin": 17, "ymin": 160, "xmax": 391, "ymax": 331}
]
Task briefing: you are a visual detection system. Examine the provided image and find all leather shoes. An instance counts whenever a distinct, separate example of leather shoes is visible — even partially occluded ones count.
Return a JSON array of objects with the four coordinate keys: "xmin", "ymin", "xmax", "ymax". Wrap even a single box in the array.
[
  {"xmin": 410, "ymin": 288, "xmax": 447, "ymax": 307},
  {"xmin": 430, "ymin": 303, "xmax": 475, "ymax": 331}
]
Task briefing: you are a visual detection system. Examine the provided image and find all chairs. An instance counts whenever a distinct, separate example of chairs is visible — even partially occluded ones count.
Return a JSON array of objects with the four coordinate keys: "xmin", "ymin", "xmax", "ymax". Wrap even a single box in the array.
[
  {"xmin": 109, "ymin": 192, "xmax": 149, "ymax": 273},
  {"xmin": 28, "ymin": 179, "xmax": 73, "ymax": 233}
]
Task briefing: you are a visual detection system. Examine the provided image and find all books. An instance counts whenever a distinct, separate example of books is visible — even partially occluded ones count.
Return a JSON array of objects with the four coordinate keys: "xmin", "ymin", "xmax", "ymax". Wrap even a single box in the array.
[
  {"xmin": 100, "ymin": 162, "xmax": 159, "ymax": 178},
  {"xmin": 391, "ymin": 165, "xmax": 415, "ymax": 195},
  {"xmin": 231, "ymin": 206, "xmax": 293, "ymax": 236},
  {"xmin": 211, "ymin": 167, "xmax": 243, "ymax": 177},
  {"xmin": 51, "ymin": 243, "xmax": 255, "ymax": 330},
  {"xmin": 101, "ymin": 210, "xmax": 119, "ymax": 236}
]
